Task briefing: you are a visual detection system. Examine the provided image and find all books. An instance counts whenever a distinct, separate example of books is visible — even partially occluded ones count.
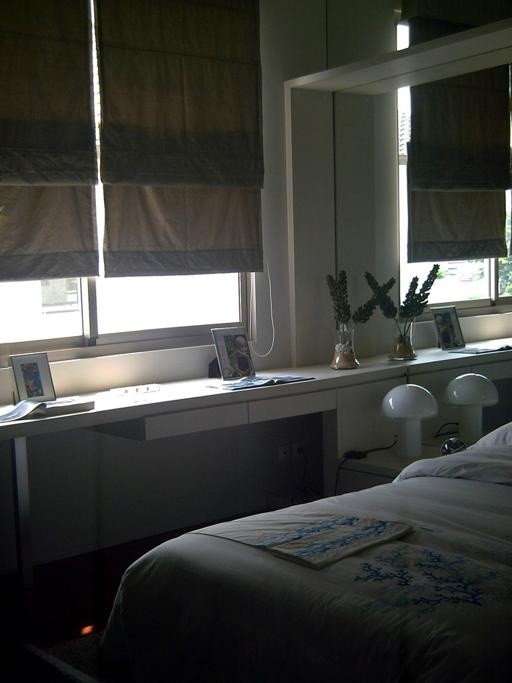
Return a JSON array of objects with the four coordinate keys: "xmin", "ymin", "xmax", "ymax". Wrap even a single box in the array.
[
  {"xmin": 205, "ymin": 373, "xmax": 316, "ymax": 390},
  {"xmin": 0, "ymin": 395, "xmax": 96, "ymax": 424},
  {"xmin": 448, "ymin": 339, "xmax": 512, "ymax": 355}
]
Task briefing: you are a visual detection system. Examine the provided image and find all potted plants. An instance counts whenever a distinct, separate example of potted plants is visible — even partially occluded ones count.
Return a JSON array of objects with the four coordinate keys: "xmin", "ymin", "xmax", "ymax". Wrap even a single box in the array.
[{"xmin": 325, "ymin": 262, "xmax": 440, "ymax": 369}]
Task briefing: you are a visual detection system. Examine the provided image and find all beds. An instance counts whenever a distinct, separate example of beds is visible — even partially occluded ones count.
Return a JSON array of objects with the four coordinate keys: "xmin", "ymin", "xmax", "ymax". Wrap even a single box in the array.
[{"xmin": 96, "ymin": 423, "xmax": 510, "ymax": 681}]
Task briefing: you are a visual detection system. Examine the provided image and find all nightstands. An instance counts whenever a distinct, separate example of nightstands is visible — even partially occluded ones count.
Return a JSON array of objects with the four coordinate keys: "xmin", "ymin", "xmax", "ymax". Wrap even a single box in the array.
[{"xmin": 335, "ymin": 441, "xmax": 442, "ymax": 494}]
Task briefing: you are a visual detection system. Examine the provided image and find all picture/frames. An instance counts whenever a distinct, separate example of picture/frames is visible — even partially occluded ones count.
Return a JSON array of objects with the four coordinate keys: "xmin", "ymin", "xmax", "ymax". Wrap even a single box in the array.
[
  {"xmin": 7, "ymin": 352, "xmax": 58, "ymax": 404},
  {"xmin": 430, "ymin": 305, "xmax": 464, "ymax": 350},
  {"xmin": 209, "ymin": 327, "xmax": 255, "ymax": 379}
]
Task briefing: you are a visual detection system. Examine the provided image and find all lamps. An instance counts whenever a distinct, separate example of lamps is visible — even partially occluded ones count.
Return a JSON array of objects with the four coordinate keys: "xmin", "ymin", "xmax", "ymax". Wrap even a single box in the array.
[{"xmin": 381, "ymin": 371, "xmax": 499, "ymax": 459}]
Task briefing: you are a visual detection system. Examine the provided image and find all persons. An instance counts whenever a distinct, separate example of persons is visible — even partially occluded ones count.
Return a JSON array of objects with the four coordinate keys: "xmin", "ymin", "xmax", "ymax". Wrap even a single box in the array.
[{"xmin": 232, "ymin": 334, "xmax": 255, "ymax": 377}]
